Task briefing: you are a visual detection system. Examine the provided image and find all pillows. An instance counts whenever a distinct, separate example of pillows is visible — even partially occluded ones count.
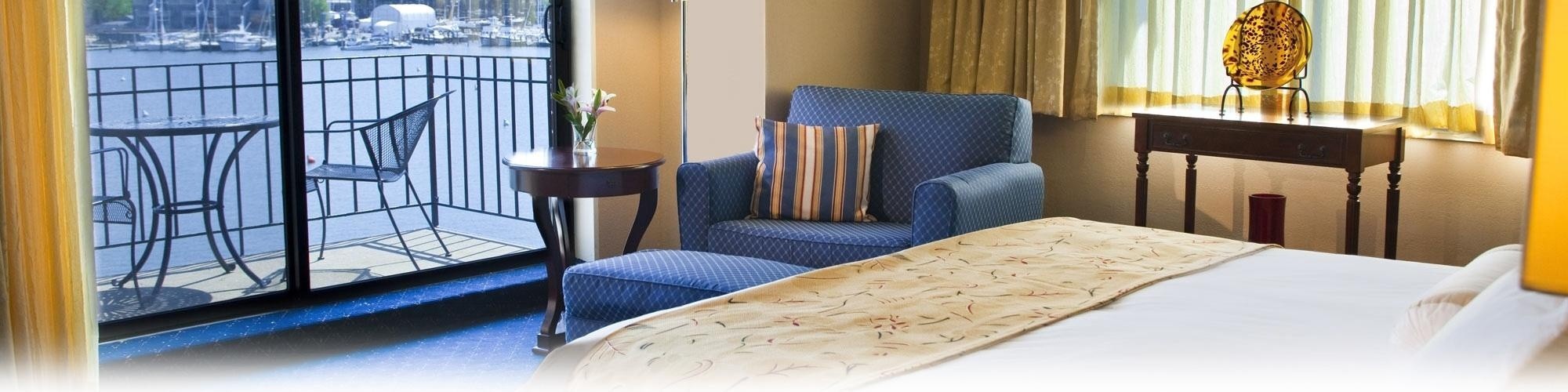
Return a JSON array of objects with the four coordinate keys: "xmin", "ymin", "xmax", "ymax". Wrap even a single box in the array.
[
  {"xmin": 749, "ymin": 112, "xmax": 879, "ymax": 227},
  {"xmin": 1312, "ymin": 242, "xmax": 1524, "ymax": 389}
]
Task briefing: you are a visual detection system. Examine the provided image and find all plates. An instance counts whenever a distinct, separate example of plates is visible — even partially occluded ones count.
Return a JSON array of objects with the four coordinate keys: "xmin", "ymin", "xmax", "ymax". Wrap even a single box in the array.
[{"xmin": 1222, "ymin": 1, "xmax": 1313, "ymax": 90}]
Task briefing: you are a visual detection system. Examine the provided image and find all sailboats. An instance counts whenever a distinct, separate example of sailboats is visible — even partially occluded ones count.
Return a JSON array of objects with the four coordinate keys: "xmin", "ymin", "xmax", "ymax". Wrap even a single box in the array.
[
  {"xmin": 301, "ymin": 0, "xmax": 551, "ymax": 51},
  {"xmin": 125, "ymin": 0, "xmax": 276, "ymax": 51}
]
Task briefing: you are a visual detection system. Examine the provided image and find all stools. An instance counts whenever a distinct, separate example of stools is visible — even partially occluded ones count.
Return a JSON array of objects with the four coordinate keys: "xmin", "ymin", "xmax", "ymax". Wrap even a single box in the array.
[{"xmin": 560, "ymin": 245, "xmax": 816, "ymax": 347}]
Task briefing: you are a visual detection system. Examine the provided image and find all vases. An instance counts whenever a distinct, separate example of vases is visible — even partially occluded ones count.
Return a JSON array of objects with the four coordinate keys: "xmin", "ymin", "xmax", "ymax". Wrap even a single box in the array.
[{"xmin": 569, "ymin": 123, "xmax": 597, "ymax": 154}]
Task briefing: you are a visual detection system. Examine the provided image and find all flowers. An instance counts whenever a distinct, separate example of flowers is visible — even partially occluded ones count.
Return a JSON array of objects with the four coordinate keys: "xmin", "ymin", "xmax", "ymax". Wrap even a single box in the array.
[{"xmin": 549, "ymin": 78, "xmax": 617, "ymax": 143}]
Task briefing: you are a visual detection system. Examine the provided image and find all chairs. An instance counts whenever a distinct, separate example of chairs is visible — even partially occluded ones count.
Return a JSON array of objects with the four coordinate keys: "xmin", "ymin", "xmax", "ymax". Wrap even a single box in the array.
[
  {"xmin": 674, "ymin": 83, "xmax": 1049, "ymax": 274},
  {"xmin": 310, "ymin": 88, "xmax": 462, "ymax": 267},
  {"xmin": 84, "ymin": 145, "xmax": 151, "ymax": 316}
]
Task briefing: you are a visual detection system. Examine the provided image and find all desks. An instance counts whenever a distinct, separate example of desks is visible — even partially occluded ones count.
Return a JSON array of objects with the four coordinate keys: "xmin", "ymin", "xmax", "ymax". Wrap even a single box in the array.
[
  {"xmin": 91, "ymin": 110, "xmax": 282, "ymax": 306},
  {"xmin": 499, "ymin": 148, "xmax": 664, "ymax": 356},
  {"xmin": 1132, "ymin": 102, "xmax": 1404, "ymax": 260}
]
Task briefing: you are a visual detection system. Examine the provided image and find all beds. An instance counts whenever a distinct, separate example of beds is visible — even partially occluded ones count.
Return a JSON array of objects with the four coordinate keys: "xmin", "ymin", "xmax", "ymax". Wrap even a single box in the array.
[{"xmin": 526, "ymin": 214, "xmax": 1463, "ymax": 392}]
